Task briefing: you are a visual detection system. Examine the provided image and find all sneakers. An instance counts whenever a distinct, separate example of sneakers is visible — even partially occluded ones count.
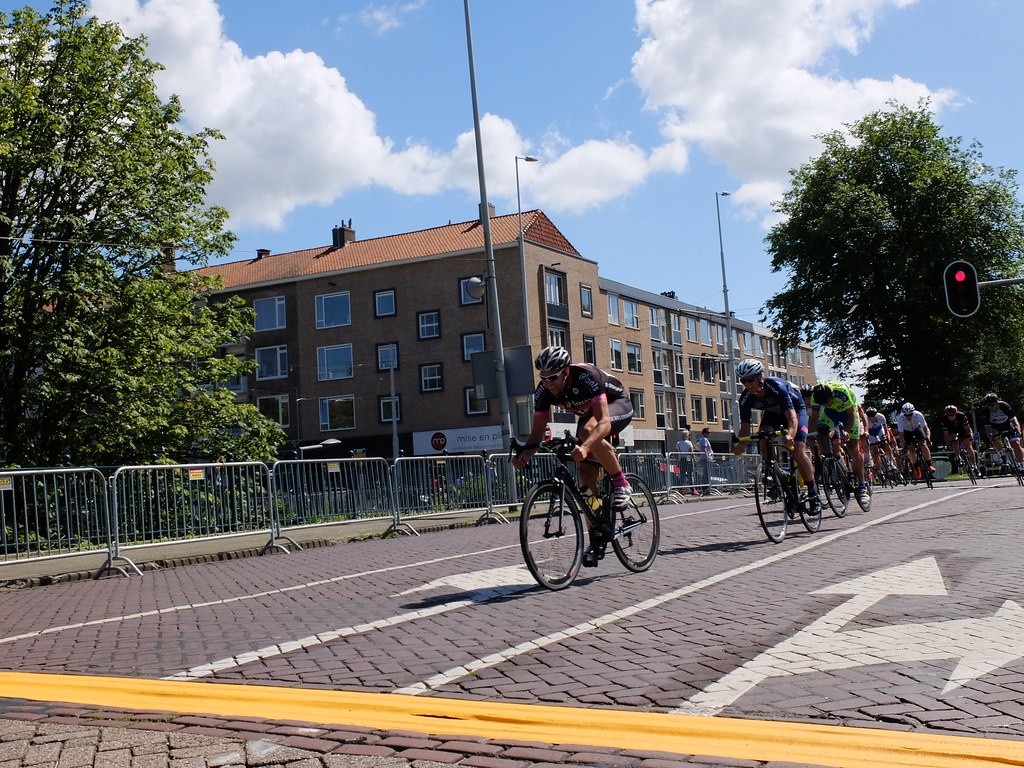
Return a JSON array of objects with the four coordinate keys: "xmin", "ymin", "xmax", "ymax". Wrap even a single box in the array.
[
  {"xmin": 613, "ymin": 484, "xmax": 633, "ymax": 507},
  {"xmin": 858, "ymin": 485, "xmax": 870, "ymax": 504},
  {"xmin": 584, "ymin": 544, "xmax": 596, "ymax": 561}
]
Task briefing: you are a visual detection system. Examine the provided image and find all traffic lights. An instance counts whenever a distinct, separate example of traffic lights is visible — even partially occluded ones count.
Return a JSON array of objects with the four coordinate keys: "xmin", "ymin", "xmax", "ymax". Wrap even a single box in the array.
[{"xmin": 943, "ymin": 258, "xmax": 983, "ymax": 318}]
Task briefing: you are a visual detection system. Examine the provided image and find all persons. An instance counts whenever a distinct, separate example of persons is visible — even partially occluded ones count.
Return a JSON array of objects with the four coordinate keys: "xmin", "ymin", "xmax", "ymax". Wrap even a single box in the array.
[
  {"xmin": 675, "ymin": 430, "xmax": 701, "ymax": 496},
  {"xmin": 940, "ymin": 405, "xmax": 982, "ymax": 477},
  {"xmin": 698, "ymin": 427, "xmax": 717, "ymax": 496},
  {"xmin": 732, "ymin": 359, "xmax": 936, "ymax": 516},
  {"xmin": 982, "ymin": 393, "xmax": 1024, "ymax": 474},
  {"xmin": 512, "ymin": 346, "xmax": 634, "ymax": 560}
]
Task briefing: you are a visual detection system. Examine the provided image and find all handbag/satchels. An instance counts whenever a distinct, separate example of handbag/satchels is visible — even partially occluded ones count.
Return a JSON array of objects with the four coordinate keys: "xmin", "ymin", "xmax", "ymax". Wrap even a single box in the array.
[{"xmin": 677, "ymin": 461, "xmax": 689, "ymax": 468}]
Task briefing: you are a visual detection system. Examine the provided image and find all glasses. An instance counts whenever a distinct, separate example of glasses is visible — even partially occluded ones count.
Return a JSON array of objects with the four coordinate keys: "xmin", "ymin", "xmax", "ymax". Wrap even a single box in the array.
[
  {"xmin": 740, "ymin": 375, "xmax": 758, "ymax": 384},
  {"xmin": 987, "ymin": 402, "xmax": 995, "ymax": 406},
  {"xmin": 538, "ymin": 368, "xmax": 564, "ymax": 381},
  {"xmin": 904, "ymin": 413, "xmax": 913, "ymax": 417}
]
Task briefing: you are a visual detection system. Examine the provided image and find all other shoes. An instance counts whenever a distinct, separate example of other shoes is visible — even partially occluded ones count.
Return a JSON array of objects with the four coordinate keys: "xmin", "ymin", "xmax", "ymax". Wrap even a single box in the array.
[
  {"xmin": 691, "ymin": 490, "xmax": 700, "ymax": 495},
  {"xmin": 1020, "ymin": 470, "xmax": 1024, "ymax": 477},
  {"xmin": 928, "ymin": 465, "xmax": 936, "ymax": 472},
  {"xmin": 889, "ymin": 461, "xmax": 897, "ymax": 470},
  {"xmin": 1001, "ymin": 455, "xmax": 1008, "ymax": 465},
  {"xmin": 913, "ymin": 467, "xmax": 923, "ymax": 479},
  {"xmin": 957, "ymin": 457, "xmax": 963, "ymax": 467},
  {"xmin": 879, "ymin": 472, "xmax": 885, "ymax": 482},
  {"xmin": 808, "ymin": 488, "xmax": 822, "ymax": 515},
  {"xmin": 974, "ymin": 468, "xmax": 981, "ymax": 477},
  {"xmin": 767, "ymin": 475, "xmax": 788, "ymax": 498}
]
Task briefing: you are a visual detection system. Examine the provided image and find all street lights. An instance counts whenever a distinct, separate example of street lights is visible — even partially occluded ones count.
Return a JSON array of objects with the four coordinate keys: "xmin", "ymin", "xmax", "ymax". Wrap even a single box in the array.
[
  {"xmin": 715, "ymin": 189, "xmax": 739, "ymax": 451},
  {"xmin": 513, "ymin": 156, "xmax": 536, "ymax": 416}
]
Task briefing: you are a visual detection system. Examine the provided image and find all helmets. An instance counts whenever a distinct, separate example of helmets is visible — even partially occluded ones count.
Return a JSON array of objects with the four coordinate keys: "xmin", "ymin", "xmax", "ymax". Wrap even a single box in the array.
[
  {"xmin": 734, "ymin": 358, "xmax": 764, "ymax": 378},
  {"xmin": 944, "ymin": 405, "xmax": 957, "ymax": 415},
  {"xmin": 812, "ymin": 384, "xmax": 833, "ymax": 405},
  {"xmin": 533, "ymin": 345, "xmax": 571, "ymax": 371},
  {"xmin": 984, "ymin": 393, "xmax": 998, "ymax": 404},
  {"xmin": 901, "ymin": 402, "xmax": 915, "ymax": 413},
  {"xmin": 866, "ymin": 406, "xmax": 878, "ymax": 417}
]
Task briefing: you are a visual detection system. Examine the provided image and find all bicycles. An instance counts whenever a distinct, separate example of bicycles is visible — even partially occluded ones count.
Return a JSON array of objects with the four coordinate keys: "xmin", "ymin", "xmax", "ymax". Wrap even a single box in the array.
[
  {"xmin": 508, "ymin": 430, "xmax": 662, "ymax": 590},
  {"xmin": 988, "ymin": 427, "xmax": 1024, "ymax": 486},
  {"xmin": 820, "ymin": 420, "xmax": 872, "ymax": 517},
  {"xmin": 905, "ymin": 423, "xmax": 934, "ymax": 490},
  {"xmin": 944, "ymin": 431, "xmax": 978, "ymax": 485},
  {"xmin": 730, "ymin": 424, "xmax": 822, "ymax": 542},
  {"xmin": 863, "ymin": 433, "xmax": 906, "ymax": 489}
]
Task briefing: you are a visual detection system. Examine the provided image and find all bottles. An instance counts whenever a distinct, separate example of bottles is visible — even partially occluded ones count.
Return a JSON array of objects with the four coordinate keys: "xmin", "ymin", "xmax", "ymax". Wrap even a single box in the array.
[
  {"xmin": 580, "ymin": 484, "xmax": 600, "ymax": 510},
  {"xmin": 836, "ymin": 456, "xmax": 848, "ymax": 471}
]
